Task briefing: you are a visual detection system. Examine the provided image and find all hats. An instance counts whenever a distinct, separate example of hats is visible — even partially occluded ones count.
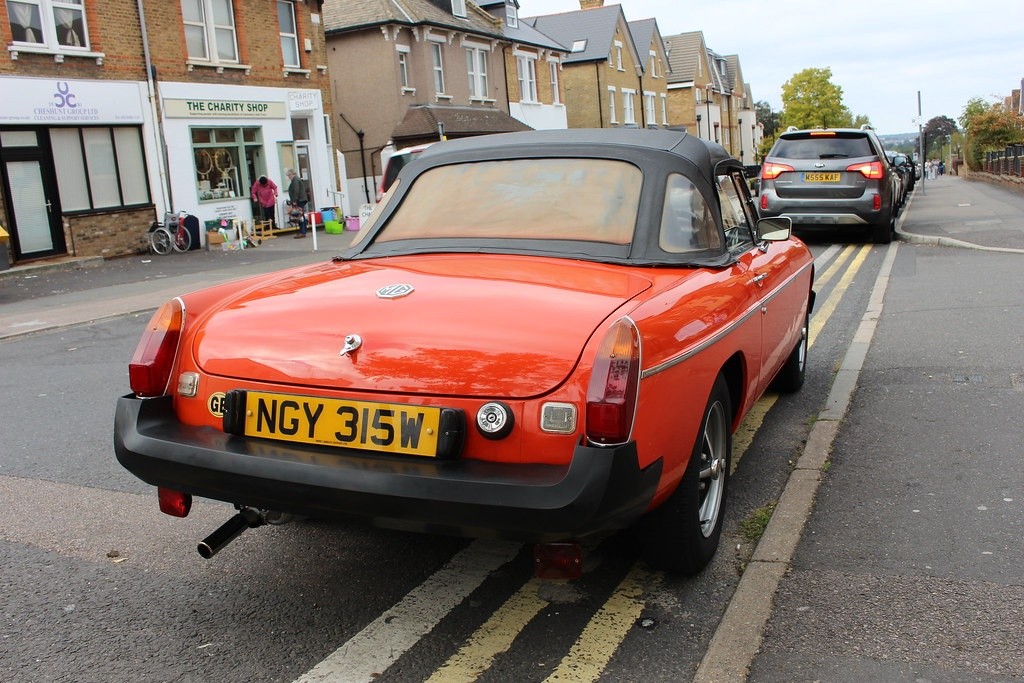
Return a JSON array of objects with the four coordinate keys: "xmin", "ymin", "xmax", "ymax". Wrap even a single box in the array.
[{"xmin": 260, "ymin": 177, "xmax": 267, "ymax": 184}]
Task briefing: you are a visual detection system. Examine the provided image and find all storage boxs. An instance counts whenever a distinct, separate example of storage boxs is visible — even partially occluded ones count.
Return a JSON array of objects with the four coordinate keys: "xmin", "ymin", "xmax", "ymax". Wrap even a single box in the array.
[
  {"xmin": 222, "ymin": 240, "xmax": 241, "ymax": 251},
  {"xmin": 205, "ymin": 233, "xmax": 230, "ymax": 244},
  {"xmin": 205, "ymin": 220, "xmax": 234, "ymax": 232},
  {"xmin": 205, "ymin": 243, "xmax": 223, "ymax": 252}
]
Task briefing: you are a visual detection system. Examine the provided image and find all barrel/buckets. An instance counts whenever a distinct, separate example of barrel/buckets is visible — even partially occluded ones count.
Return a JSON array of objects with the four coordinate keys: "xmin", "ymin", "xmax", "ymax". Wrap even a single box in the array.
[
  {"xmin": 321, "ymin": 210, "xmax": 333, "ymax": 223},
  {"xmin": 324, "ymin": 221, "xmax": 339, "ymax": 234},
  {"xmin": 308, "ymin": 211, "xmax": 321, "ymax": 225},
  {"xmin": 345, "ymin": 217, "xmax": 359, "ymax": 231},
  {"xmin": 333, "ymin": 224, "xmax": 343, "ymax": 234}
]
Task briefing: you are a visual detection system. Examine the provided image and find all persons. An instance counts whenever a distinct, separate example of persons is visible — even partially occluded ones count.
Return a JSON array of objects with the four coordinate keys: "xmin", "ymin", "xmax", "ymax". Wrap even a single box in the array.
[
  {"xmin": 925, "ymin": 156, "xmax": 941, "ymax": 180},
  {"xmin": 252, "ymin": 177, "xmax": 278, "ymax": 230},
  {"xmin": 286, "ymin": 169, "xmax": 308, "ymax": 239}
]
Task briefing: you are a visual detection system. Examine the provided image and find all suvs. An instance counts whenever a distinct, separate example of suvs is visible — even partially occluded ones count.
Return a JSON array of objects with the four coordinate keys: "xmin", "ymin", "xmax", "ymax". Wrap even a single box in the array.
[{"xmin": 756, "ymin": 125, "xmax": 909, "ymax": 243}]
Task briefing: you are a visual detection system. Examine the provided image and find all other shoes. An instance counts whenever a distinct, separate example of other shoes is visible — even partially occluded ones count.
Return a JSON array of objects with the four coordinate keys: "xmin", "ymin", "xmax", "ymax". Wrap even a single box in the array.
[{"xmin": 294, "ymin": 233, "xmax": 306, "ymax": 238}]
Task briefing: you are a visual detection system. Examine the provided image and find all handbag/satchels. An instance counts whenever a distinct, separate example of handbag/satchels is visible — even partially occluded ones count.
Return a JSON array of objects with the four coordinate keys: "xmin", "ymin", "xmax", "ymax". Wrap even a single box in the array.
[{"xmin": 287, "ymin": 206, "xmax": 302, "ymax": 227}]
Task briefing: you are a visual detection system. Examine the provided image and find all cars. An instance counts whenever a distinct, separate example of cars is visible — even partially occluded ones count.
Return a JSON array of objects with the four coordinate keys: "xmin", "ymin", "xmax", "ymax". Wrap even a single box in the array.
[
  {"xmin": 884, "ymin": 150, "xmax": 921, "ymax": 208},
  {"xmin": 113, "ymin": 127, "xmax": 816, "ymax": 579}
]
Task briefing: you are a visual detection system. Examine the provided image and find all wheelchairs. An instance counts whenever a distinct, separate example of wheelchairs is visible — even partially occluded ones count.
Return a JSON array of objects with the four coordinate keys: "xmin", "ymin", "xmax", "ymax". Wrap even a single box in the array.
[{"xmin": 145, "ymin": 210, "xmax": 191, "ymax": 255}]
